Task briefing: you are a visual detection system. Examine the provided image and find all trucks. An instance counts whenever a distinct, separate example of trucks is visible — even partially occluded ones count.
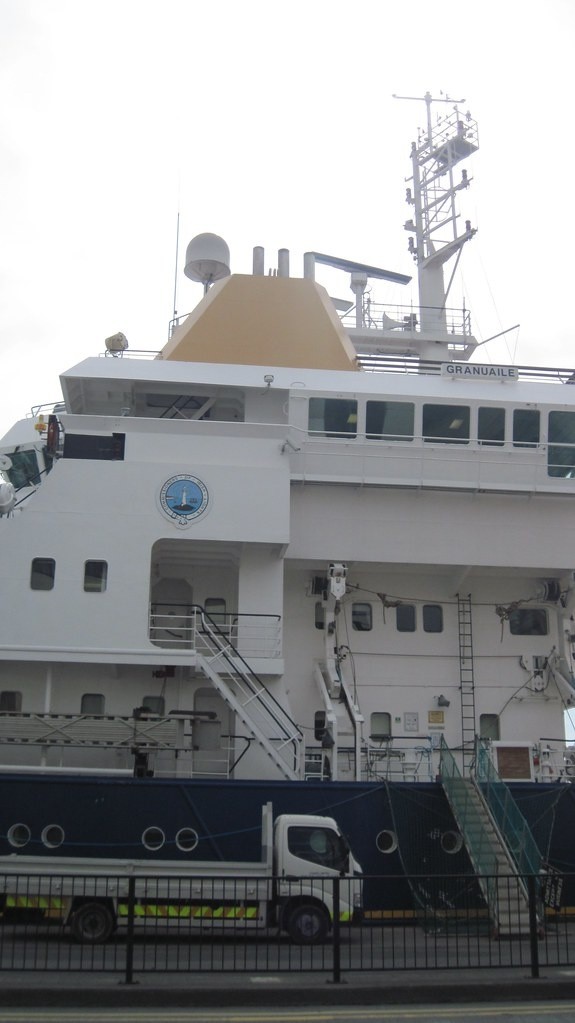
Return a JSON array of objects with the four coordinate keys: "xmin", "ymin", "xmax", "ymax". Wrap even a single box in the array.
[{"xmin": 0, "ymin": 801, "xmax": 364, "ymax": 945}]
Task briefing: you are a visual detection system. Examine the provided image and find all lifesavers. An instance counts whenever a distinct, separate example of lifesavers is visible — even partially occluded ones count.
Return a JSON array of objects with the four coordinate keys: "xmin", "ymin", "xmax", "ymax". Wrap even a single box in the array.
[{"xmin": 535, "ymin": 762, "xmax": 556, "ymax": 784}]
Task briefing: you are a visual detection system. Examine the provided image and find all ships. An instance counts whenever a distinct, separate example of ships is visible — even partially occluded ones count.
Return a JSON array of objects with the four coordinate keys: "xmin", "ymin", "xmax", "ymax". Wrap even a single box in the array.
[{"xmin": 1, "ymin": 89, "xmax": 574, "ymax": 940}]
